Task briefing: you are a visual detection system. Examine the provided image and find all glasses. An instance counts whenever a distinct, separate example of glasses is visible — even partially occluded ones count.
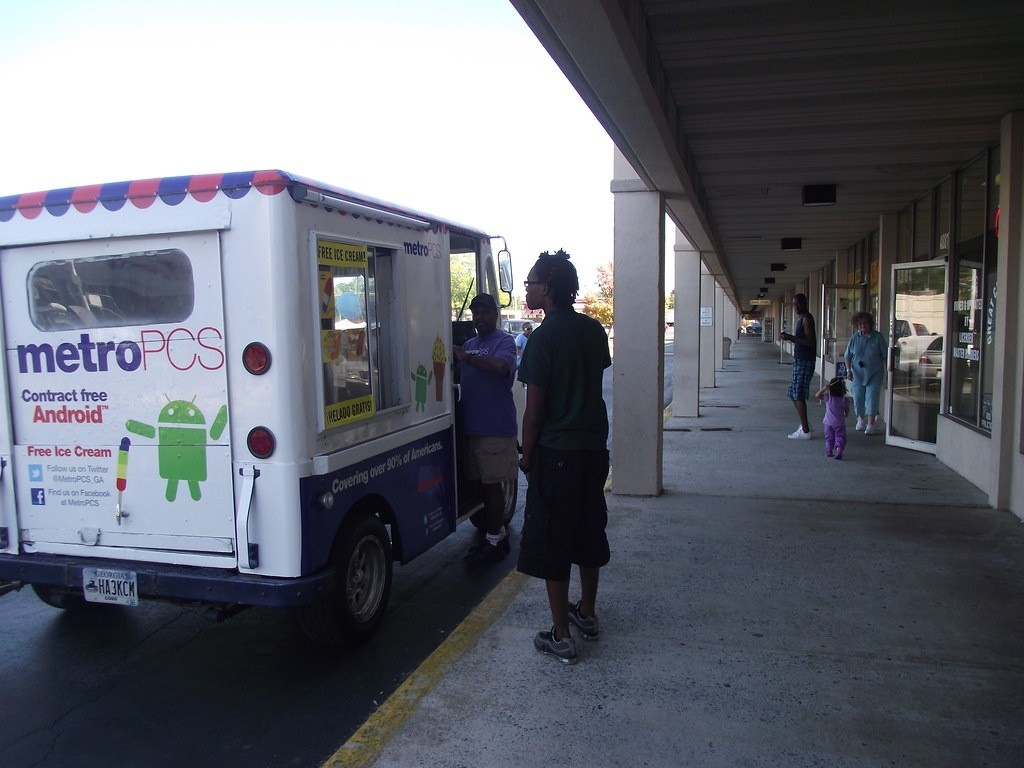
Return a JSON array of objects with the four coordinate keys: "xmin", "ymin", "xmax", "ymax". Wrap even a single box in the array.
[
  {"xmin": 524, "ymin": 328, "xmax": 533, "ymax": 331},
  {"xmin": 524, "ymin": 280, "xmax": 545, "ymax": 287}
]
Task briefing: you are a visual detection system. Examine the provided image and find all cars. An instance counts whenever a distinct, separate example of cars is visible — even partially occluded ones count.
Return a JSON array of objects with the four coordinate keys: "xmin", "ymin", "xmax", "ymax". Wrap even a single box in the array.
[
  {"xmin": 501, "ymin": 318, "xmax": 540, "ymax": 344},
  {"xmin": 747, "ymin": 323, "xmax": 761, "ymax": 334},
  {"xmin": 892, "ymin": 318, "xmax": 945, "ymax": 389}
]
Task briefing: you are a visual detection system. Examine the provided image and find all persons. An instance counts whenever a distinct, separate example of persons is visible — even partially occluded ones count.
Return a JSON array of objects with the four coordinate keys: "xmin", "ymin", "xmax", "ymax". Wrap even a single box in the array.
[
  {"xmin": 450, "ymin": 293, "xmax": 517, "ymax": 571},
  {"xmin": 779, "ymin": 294, "xmax": 817, "ymax": 440},
  {"xmin": 519, "ymin": 248, "xmax": 612, "ymax": 665},
  {"xmin": 815, "ymin": 378, "xmax": 849, "ymax": 459},
  {"xmin": 515, "ymin": 322, "xmax": 533, "ymax": 363},
  {"xmin": 844, "ymin": 311, "xmax": 888, "ymax": 434}
]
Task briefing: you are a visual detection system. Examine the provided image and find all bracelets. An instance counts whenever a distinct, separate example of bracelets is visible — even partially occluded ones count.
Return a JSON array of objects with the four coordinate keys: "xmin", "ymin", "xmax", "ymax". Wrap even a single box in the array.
[{"xmin": 466, "ymin": 352, "xmax": 474, "ymax": 364}]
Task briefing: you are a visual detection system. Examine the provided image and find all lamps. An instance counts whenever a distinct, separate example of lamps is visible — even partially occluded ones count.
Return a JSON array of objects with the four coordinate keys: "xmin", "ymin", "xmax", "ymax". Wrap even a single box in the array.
[
  {"xmin": 781, "ymin": 238, "xmax": 802, "ymax": 251},
  {"xmin": 802, "ymin": 184, "xmax": 836, "ymax": 207},
  {"xmin": 771, "ymin": 263, "xmax": 788, "ymax": 272},
  {"xmin": 765, "ymin": 278, "xmax": 775, "ymax": 284},
  {"xmin": 760, "ymin": 288, "xmax": 768, "ymax": 292}
]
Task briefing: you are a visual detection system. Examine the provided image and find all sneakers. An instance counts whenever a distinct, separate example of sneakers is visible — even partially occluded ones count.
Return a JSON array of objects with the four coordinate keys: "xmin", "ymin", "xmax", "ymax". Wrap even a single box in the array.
[
  {"xmin": 787, "ymin": 423, "xmax": 815, "ymax": 440},
  {"xmin": 533, "ymin": 625, "xmax": 579, "ymax": 665},
  {"xmin": 568, "ymin": 600, "xmax": 599, "ymax": 642}
]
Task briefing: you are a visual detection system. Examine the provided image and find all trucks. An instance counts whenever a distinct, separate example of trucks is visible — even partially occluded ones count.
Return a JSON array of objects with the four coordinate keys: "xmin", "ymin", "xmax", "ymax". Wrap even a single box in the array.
[{"xmin": 0, "ymin": 168, "xmax": 517, "ymax": 639}]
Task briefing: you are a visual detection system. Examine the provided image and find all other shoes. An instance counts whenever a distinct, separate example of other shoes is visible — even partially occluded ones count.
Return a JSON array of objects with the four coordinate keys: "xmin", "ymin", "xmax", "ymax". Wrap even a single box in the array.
[
  {"xmin": 463, "ymin": 536, "xmax": 511, "ymax": 570},
  {"xmin": 825, "ymin": 449, "xmax": 832, "ymax": 457},
  {"xmin": 835, "ymin": 448, "xmax": 843, "ymax": 459},
  {"xmin": 855, "ymin": 418, "xmax": 865, "ymax": 430},
  {"xmin": 865, "ymin": 423, "xmax": 875, "ymax": 435}
]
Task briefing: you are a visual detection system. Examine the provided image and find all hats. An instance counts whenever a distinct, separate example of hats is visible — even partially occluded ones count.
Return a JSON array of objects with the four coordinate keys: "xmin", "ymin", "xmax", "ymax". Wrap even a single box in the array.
[{"xmin": 469, "ymin": 293, "xmax": 497, "ymax": 312}]
